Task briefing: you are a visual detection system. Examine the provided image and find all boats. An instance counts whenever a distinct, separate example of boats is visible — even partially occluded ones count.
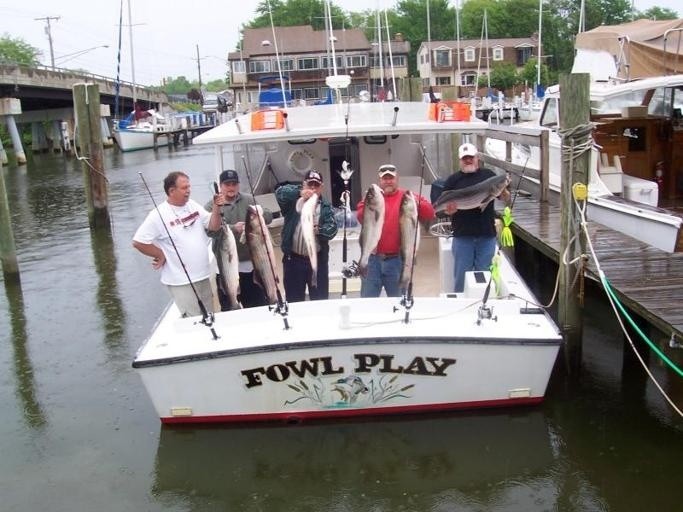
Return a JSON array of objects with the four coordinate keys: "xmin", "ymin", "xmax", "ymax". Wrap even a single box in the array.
[
  {"xmin": 110, "ymin": 109, "xmax": 209, "ymax": 153},
  {"xmin": 569, "ymin": 15, "xmax": 682, "ymax": 86},
  {"xmin": 483, "ymin": 73, "xmax": 682, "ymax": 254},
  {"xmin": 453, "ymin": 1, "xmax": 584, "ymax": 121},
  {"xmin": 128, "ymin": 75, "xmax": 566, "ymax": 428}
]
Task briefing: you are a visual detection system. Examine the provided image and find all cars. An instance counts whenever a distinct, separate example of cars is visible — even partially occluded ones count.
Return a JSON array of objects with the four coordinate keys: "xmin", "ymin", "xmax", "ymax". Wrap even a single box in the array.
[{"xmin": 202, "ymin": 94, "xmax": 233, "ymax": 112}]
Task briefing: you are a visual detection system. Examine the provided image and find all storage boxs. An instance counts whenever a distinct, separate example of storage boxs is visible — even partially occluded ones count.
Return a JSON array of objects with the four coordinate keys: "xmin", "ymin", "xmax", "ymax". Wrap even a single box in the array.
[{"xmin": 622, "ymin": 172, "xmax": 659, "ymax": 207}]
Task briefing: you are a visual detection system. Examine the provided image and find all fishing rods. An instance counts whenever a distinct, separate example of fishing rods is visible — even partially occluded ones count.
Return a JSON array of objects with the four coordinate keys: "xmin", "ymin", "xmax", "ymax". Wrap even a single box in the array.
[
  {"xmin": 339, "ymin": 117, "xmax": 354, "ymax": 298},
  {"xmin": 476, "ymin": 155, "xmax": 530, "ymax": 325},
  {"xmin": 400, "ymin": 146, "xmax": 426, "ymax": 324},
  {"xmin": 140, "ymin": 170, "xmax": 217, "ymax": 339},
  {"xmin": 241, "ymin": 155, "xmax": 289, "ymax": 330}
]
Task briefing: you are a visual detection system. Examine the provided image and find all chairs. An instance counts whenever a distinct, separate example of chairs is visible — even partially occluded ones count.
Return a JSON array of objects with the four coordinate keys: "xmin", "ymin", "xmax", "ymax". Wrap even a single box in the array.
[{"xmin": 252, "ymin": 192, "xmax": 285, "ymax": 247}]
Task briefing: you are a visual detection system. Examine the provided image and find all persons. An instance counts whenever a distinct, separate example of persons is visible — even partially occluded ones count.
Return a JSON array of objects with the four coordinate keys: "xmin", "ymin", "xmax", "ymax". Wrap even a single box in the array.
[
  {"xmin": 436, "ymin": 143, "xmax": 512, "ymax": 293},
  {"xmin": 357, "ymin": 163, "xmax": 436, "ymax": 298},
  {"xmin": 202, "ymin": 170, "xmax": 272, "ymax": 311},
  {"xmin": 133, "ymin": 172, "xmax": 225, "ymax": 318},
  {"xmin": 274, "ymin": 170, "xmax": 339, "ymax": 302}
]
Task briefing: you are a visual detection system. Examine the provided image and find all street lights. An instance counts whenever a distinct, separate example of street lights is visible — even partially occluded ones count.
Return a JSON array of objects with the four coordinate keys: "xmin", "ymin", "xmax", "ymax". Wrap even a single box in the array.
[
  {"xmin": 261, "ymin": 38, "xmax": 287, "ymax": 108},
  {"xmin": 51, "ymin": 44, "xmax": 109, "ymax": 69},
  {"xmin": 326, "ymin": 36, "xmax": 342, "ymax": 103}
]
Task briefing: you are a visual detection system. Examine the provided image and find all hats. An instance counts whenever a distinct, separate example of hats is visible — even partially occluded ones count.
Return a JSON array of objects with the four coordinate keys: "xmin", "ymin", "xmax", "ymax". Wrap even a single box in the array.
[
  {"xmin": 458, "ymin": 144, "xmax": 477, "ymax": 159},
  {"xmin": 378, "ymin": 165, "xmax": 396, "ymax": 178},
  {"xmin": 220, "ymin": 170, "xmax": 238, "ymax": 183},
  {"xmin": 305, "ymin": 170, "xmax": 322, "ymax": 185}
]
